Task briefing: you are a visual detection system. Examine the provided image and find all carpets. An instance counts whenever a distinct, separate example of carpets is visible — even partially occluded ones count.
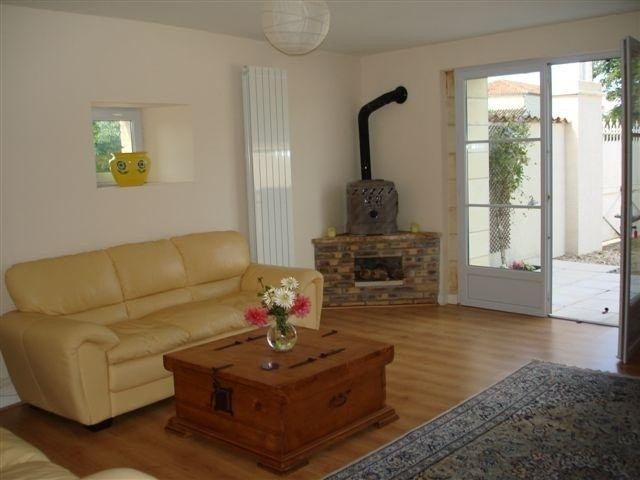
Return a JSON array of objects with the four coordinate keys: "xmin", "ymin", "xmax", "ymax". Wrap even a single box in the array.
[{"xmin": 318, "ymin": 358, "xmax": 639, "ymax": 480}]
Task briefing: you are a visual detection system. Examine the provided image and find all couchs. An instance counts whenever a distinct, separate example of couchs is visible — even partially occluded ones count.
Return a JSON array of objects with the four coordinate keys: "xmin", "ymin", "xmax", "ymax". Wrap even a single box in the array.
[{"xmin": 0, "ymin": 229, "xmax": 324, "ymax": 433}]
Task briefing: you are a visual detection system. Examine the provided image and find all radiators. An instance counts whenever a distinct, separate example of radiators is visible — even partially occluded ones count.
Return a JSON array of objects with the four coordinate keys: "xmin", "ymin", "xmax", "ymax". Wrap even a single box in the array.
[{"xmin": 241, "ymin": 64, "xmax": 295, "ymax": 270}]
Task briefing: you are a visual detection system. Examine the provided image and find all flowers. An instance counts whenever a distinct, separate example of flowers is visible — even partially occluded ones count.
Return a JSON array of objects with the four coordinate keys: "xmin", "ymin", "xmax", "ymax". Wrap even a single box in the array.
[
  {"xmin": 506, "ymin": 260, "xmax": 536, "ymax": 271},
  {"xmin": 244, "ymin": 274, "xmax": 312, "ymax": 347}
]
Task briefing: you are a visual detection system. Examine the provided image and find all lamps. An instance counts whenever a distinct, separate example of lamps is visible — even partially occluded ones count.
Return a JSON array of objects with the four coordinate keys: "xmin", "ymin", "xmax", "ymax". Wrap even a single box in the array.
[{"xmin": 261, "ymin": 2, "xmax": 330, "ymax": 55}]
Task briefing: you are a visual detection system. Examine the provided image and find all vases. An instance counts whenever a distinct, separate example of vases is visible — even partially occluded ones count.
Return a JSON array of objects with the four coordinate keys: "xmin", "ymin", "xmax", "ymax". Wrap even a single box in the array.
[
  {"xmin": 267, "ymin": 311, "xmax": 298, "ymax": 352},
  {"xmin": 109, "ymin": 152, "xmax": 150, "ymax": 187}
]
